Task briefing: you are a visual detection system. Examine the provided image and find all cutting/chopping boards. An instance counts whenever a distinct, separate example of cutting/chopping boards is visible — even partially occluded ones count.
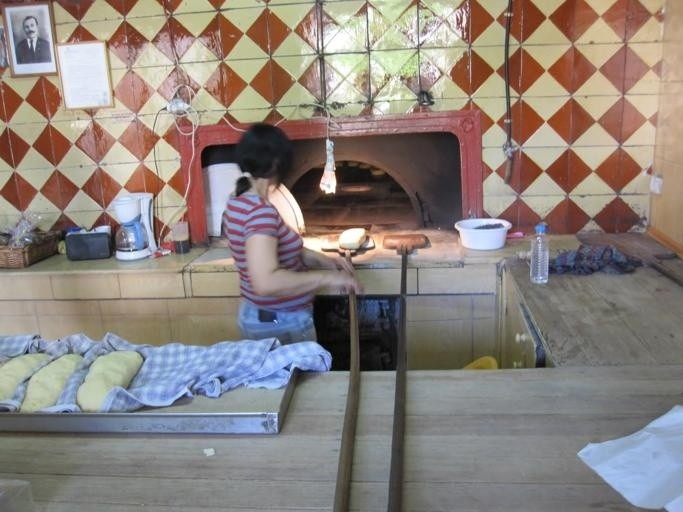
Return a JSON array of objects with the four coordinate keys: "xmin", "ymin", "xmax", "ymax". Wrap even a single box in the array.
[
  {"xmin": 382, "ymin": 234, "xmax": 429, "ymax": 255},
  {"xmin": 321, "ymin": 238, "xmax": 374, "ymax": 253},
  {"xmin": 574, "ymin": 230, "xmax": 677, "ymax": 266}
]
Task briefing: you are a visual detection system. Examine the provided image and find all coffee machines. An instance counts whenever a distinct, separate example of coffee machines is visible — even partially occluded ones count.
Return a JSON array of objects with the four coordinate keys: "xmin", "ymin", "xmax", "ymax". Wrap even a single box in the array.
[{"xmin": 108, "ymin": 190, "xmax": 159, "ymax": 261}]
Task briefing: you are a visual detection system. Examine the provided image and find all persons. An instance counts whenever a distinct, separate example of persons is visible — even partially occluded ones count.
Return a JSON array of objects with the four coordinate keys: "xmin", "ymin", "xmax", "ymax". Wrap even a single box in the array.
[
  {"xmin": 223, "ymin": 123, "xmax": 361, "ymax": 345},
  {"xmin": 16, "ymin": 16, "xmax": 51, "ymax": 64}
]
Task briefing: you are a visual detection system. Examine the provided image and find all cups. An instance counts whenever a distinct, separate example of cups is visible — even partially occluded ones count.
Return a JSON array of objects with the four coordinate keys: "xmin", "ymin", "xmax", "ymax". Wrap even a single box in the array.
[{"xmin": 169, "ymin": 221, "xmax": 191, "ymax": 255}]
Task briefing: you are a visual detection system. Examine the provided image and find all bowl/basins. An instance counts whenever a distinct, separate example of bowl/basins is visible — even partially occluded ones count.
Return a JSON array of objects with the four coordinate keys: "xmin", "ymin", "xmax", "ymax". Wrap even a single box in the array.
[{"xmin": 453, "ymin": 217, "xmax": 512, "ymax": 251}]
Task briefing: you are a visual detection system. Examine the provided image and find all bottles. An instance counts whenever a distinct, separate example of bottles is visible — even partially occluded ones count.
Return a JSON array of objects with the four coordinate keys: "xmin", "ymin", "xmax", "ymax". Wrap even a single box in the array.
[{"xmin": 528, "ymin": 224, "xmax": 549, "ymax": 285}]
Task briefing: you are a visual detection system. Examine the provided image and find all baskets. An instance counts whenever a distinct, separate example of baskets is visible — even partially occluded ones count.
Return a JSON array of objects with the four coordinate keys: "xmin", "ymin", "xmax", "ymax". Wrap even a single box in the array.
[{"xmin": 0, "ymin": 231, "xmax": 58, "ymax": 268}]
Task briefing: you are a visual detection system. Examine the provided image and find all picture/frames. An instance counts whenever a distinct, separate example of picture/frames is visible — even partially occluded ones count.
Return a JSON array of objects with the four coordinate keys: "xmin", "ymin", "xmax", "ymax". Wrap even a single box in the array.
[{"xmin": 0, "ymin": 1, "xmax": 58, "ymax": 80}]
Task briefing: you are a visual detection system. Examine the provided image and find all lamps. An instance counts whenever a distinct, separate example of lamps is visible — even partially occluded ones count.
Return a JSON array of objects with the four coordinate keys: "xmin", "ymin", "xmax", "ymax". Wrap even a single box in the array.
[{"xmin": 319, "ymin": 130, "xmax": 340, "ymax": 194}]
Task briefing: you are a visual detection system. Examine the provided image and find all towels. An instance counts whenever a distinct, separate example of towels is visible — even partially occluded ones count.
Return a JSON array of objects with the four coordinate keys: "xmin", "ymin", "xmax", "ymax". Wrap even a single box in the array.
[{"xmin": 547, "ymin": 239, "xmax": 642, "ymax": 278}]
